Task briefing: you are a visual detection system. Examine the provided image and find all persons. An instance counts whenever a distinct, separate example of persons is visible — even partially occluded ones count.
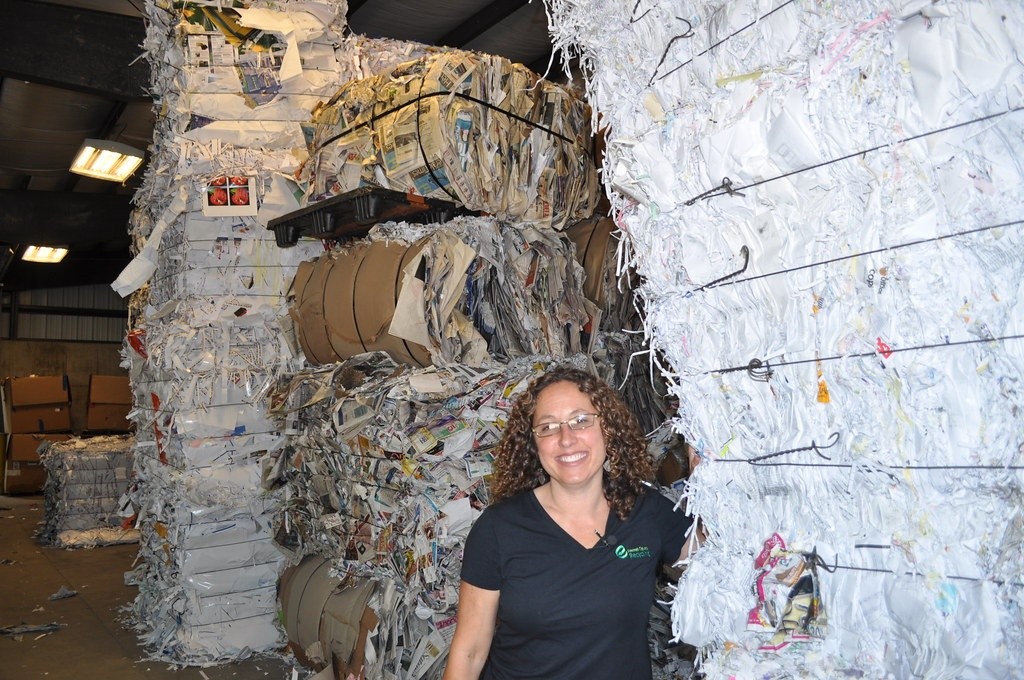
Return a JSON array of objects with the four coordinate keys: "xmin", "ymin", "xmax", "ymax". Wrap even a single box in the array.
[{"xmin": 443, "ymin": 366, "xmax": 706, "ymax": 680}]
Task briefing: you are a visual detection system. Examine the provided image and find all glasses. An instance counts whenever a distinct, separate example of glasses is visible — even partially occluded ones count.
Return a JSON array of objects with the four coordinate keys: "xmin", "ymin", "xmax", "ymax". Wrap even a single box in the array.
[{"xmin": 531, "ymin": 413, "xmax": 601, "ymax": 438}]
